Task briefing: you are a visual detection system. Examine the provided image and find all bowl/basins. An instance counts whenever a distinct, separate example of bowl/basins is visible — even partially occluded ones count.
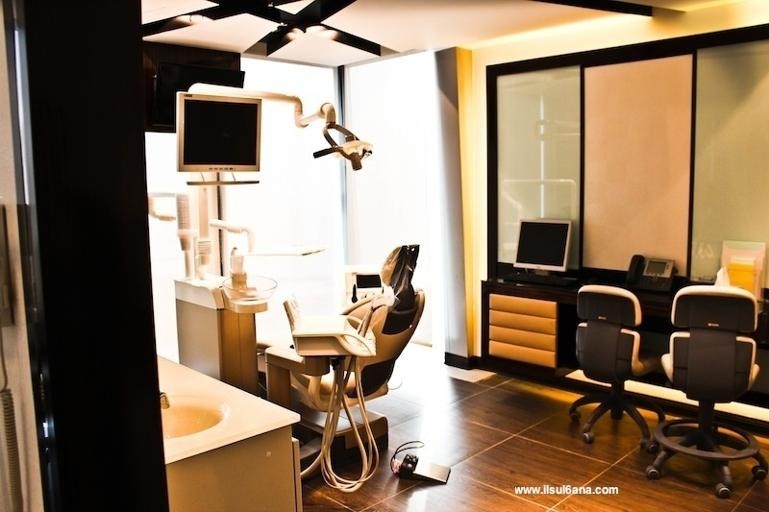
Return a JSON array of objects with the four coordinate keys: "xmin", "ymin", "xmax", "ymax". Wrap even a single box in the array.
[{"xmin": 224, "ymin": 275, "xmax": 277, "ymax": 302}]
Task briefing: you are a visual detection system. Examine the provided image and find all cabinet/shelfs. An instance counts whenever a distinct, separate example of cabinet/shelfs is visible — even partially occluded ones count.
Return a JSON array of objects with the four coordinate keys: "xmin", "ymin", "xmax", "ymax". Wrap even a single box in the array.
[{"xmin": 481, "ymin": 280, "xmax": 769, "ymax": 435}]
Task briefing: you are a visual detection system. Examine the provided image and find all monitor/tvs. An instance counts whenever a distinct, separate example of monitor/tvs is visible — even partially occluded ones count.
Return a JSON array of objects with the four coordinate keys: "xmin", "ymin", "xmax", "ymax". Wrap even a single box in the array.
[
  {"xmin": 175, "ymin": 91, "xmax": 262, "ymax": 173},
  {"xmin": 512, "ymin": 216, "xmax": 572, "ymax": 276}
]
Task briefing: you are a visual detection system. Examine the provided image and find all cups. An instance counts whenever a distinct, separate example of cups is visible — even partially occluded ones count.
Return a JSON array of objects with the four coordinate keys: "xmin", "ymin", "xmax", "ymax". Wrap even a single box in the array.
[{"xmin": 230, "ymin": 272, "xmax": 247, "ymax": 290}]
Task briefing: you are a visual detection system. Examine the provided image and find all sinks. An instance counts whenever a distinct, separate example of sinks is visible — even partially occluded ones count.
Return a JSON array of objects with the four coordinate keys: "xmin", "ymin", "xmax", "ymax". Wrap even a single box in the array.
[{"xmin": 161, "ymin": 393, "xmax": 230, "ymax": 441}]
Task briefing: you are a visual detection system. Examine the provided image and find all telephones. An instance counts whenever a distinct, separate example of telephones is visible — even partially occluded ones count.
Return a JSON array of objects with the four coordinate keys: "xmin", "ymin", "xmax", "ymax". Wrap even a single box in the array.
[{"xmin": 625, "ymin": 255, "xmax": 676, "ymax": 294}]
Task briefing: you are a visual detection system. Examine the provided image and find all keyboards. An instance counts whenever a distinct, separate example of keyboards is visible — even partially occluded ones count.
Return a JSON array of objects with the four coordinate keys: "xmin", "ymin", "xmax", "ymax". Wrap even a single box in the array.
[{"xmin": 504, "ymin": 273, "xmax": 578, "ymax": 287}]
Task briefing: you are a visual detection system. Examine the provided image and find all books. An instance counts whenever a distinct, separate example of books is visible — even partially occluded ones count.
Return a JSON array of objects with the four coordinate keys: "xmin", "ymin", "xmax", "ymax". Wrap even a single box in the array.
[{"xmin": 721, "ymin": 240, "xmax": 765, "ymax": 301}]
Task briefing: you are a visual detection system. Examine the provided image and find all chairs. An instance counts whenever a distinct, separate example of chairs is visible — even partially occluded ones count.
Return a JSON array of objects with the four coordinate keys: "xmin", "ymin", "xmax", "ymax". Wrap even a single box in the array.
[
  {"xmin": 568, "ymin": 285, "xmax": 667, "ymax": 454},
  {"xmin": 646, "ymin": 285, "xmax": 769, "ymax": 498},
  {"xmin": 256, "ymin": 244, "xmax": 425, "ymax": 413}
]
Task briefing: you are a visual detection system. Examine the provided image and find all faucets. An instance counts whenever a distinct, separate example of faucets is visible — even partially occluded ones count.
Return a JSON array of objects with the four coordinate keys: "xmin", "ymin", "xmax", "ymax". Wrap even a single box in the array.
[{"xmin": 159, "ymin": 391, "xmax": 170, "ymax": 409}]
